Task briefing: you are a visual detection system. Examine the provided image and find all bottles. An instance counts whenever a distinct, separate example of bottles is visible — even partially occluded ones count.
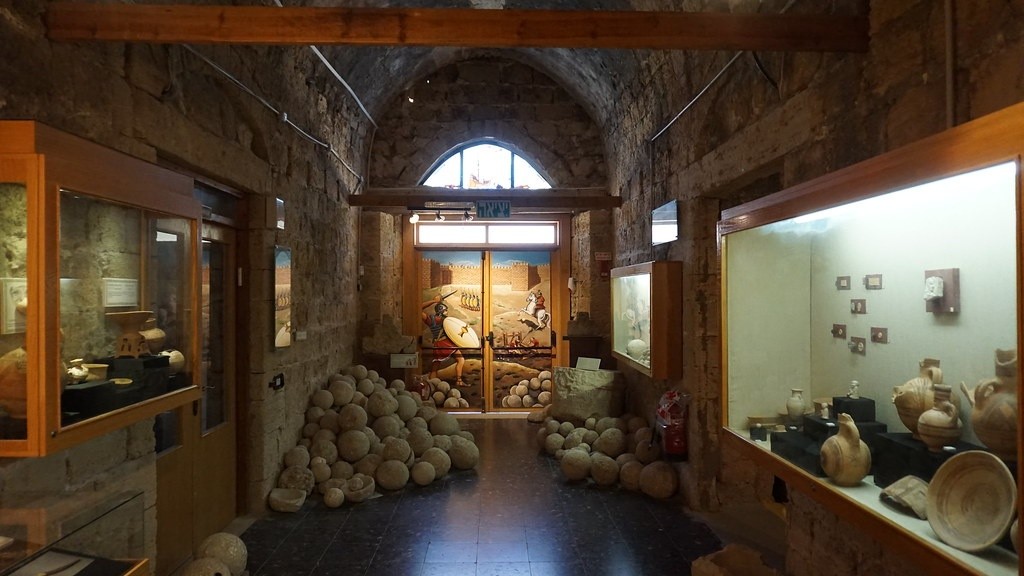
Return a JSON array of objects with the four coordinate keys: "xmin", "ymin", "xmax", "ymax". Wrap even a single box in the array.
[
  {"xmin": 139, "ymin": 318, "xmax": 166, "ymax": 351},
  {"xmin": 960, "ymin": 348, "xmax": 1017, "ymax": 452},
  {"xmin": 67, "ymin": 359, "xmax": 89, "ymax": 384},
  {"xmin": 821, "ymin": 413, "xmax": 871, "ymax": 486},
  {"xmin": 787, "ymin": 389, "xmax": 805, "ymax": 421},
  {"xmin": 918, "ymin": 384, "xmax": 963, "ymax": 452},
  {"xmin": 891, "ymin": 358, "xmax": 960, "ymax": 440},
  {"xmin": 0, "ymin": 347, "xmax": 67, "ymax": 419}
]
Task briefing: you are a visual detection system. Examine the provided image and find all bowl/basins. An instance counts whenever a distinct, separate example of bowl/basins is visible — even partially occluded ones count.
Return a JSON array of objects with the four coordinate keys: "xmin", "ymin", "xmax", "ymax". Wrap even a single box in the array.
[
  {"xmin": 110, "ymin": 379, "xmax": 132, "ymax": 388},
  {"xmin": 82, "ymin": 364, "xmax": 108, "ymax": 380},
  {"xmin": 926, "ymin": 451, "xmax": 1017, "ymax": 551},
  {"xmin": 748, "ymin": 417, "xmax": 783, "ymax": 432}
]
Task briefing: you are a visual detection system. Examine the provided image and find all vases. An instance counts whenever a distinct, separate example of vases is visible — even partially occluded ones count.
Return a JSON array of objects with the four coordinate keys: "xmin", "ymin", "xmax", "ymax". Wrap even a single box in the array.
[{"xmin": 787, "ymin": 388, "xmax": 805, "ymax": 421}]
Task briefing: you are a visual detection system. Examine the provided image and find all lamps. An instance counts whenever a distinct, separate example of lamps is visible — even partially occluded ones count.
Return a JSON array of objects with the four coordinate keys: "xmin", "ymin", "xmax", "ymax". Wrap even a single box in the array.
[
  {"xmin": 435, "ymin": 208, "xmax": 446, "ymax": 223},
  {"xmin": 461, "ymin": 211, "xmax": 473, "ymax": 222},
  {"xmin": 406, "ymin": 210, "xmax": 420, "ymax": 224}
]
